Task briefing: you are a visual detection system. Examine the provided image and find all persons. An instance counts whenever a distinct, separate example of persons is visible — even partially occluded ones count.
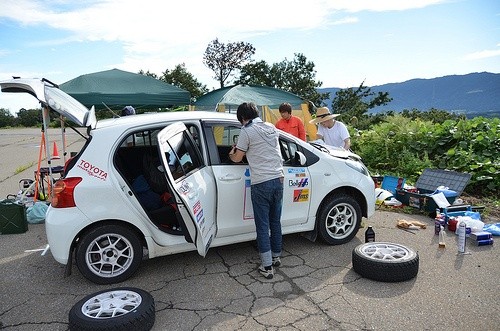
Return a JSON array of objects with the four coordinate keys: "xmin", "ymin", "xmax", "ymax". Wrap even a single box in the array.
[
  {"xmin": 227, "ymin": 101, "xmax": 284, "ymax": 279},
  {"xmin": 315, "ymin": 106, "xmax": 351, "ymax": 151},
  {"xmin": 275, "ymin": 102, "xmax": 307, "ymax": 144}
]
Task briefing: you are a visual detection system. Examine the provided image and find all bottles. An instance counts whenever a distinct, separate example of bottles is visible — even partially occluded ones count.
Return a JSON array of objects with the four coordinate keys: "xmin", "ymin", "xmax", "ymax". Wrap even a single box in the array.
[
  {"xmin": 457, "ymin": 220, "xmax": 466, "ymax": 252},
  {"xmin": 439, "ymin": 227, "xmax": 446, "ymax": 248},
  {"xmin": 435, "ymin": 216, "xmax": 441, "ymax": 235},
  {"xmin": 364, "ymin": 226, "xmax": 375, "ymax": 243}
]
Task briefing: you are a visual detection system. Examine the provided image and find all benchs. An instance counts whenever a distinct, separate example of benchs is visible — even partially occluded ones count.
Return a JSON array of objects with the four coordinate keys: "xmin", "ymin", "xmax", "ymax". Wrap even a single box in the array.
[{"xmin": 115, "ymin": 148, "xmax": 177, "ymax": 226}]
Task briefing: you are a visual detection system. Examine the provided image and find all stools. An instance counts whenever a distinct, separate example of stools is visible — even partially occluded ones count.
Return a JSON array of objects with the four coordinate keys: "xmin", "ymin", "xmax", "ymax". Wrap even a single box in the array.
[{"xmin": 34, "ymin": 166, "xmax": 65, "ymax": 199}]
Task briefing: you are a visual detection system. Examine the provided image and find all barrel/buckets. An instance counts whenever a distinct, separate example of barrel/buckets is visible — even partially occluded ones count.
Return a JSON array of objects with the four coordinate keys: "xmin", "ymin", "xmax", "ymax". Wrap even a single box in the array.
[{"xmin": 0, "ymin": 199, "xmax": 28, "ymax": 234}]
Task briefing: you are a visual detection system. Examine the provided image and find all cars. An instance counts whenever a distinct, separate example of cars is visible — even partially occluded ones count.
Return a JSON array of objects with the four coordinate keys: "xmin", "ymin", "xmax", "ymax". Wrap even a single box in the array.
[{"xmin": 0, "ymin": 77, "xmax": 377, "ymax": 285}]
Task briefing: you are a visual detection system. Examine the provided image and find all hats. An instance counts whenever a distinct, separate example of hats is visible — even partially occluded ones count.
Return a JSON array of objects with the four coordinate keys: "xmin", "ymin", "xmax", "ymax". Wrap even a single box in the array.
[
  {"xmin": 122, "ymin": 106, "xmax": 135, "ymax": 115},
  {"xmin": 309, "ymin": 107, "xmax": 341, "ymax": 125}
]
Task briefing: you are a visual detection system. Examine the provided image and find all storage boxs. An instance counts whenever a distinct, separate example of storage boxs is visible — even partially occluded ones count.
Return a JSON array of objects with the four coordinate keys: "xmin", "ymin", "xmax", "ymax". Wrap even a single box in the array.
[{"xmin": 394, "ymin": 167, "xmax": 472, "ymax": 211}]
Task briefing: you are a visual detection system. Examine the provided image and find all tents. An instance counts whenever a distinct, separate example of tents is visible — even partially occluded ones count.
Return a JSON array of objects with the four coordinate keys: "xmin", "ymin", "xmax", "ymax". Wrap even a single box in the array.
[
  {"xmin": 41, "ymin": 67, "xmax": 191, "ymax": 196},
  {"xmin": 188, "ymin": 82, "xmax": 319, "ymax": 142}
]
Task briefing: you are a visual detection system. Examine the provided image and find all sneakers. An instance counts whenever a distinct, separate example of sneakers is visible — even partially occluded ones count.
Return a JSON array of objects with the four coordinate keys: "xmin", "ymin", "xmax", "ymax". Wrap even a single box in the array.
[
  {"xmin": 272, "ymin": 257, "xmax": 281, "ymax": 266},
  {"xmin": 258, "ymin": 266, "xmax": 273, "ymax": 280}
]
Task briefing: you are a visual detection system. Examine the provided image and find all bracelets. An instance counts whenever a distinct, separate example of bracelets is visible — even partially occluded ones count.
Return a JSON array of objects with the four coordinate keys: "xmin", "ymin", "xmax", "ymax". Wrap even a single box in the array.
[{"xmin": 228, "ymin": 154, "xmax": 232, "ymax": 157}]
